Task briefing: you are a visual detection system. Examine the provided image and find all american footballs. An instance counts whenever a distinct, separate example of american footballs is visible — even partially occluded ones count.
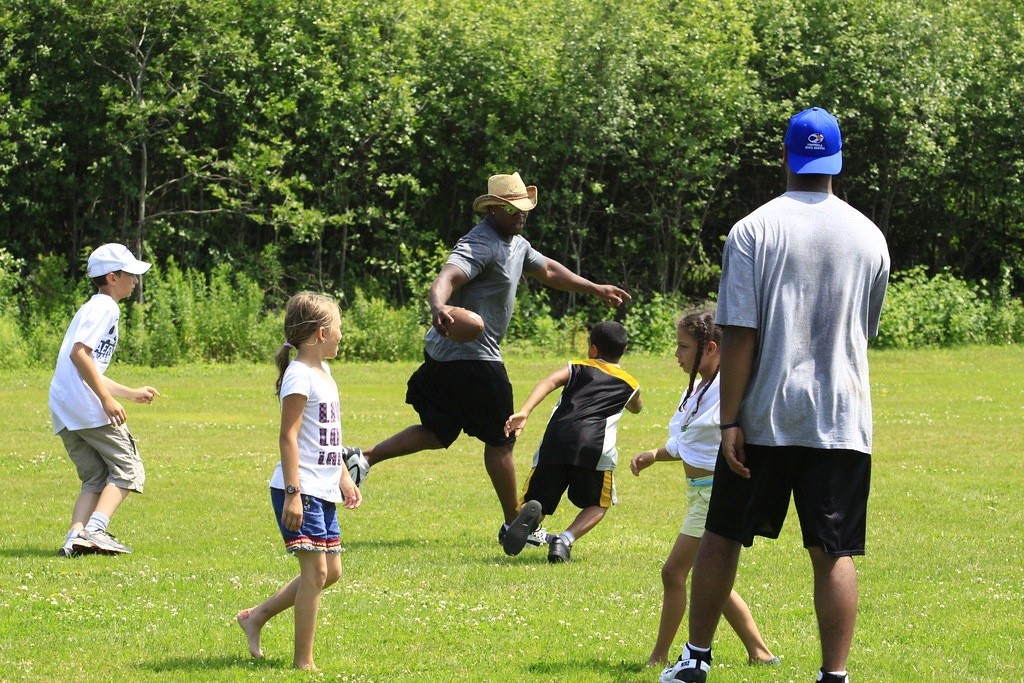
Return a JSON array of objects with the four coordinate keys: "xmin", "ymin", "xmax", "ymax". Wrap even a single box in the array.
[{"xmin": 444, "ymin": 309, "xmax": 484, "ymax": 340}]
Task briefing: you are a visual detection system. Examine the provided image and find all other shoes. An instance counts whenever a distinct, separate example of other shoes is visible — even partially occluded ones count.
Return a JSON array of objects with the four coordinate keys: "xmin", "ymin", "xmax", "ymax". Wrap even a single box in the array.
[{"xmin": 719, "ymin": 656, "xmax": 781, "ymax": 668}]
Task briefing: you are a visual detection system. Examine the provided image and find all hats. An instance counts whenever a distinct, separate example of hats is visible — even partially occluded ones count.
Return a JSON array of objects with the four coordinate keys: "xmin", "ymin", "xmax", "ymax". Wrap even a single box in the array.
[
  {"xmin": 785, "ymin": 107, "xmax": 842, "ymax": 175},
  {"xmin": 87, "ymin": 243, "xmax": 152, "ymax": 278},
  {"xmin": 473, "ymin": 172, "xmax": 538, "ymax": 214}
]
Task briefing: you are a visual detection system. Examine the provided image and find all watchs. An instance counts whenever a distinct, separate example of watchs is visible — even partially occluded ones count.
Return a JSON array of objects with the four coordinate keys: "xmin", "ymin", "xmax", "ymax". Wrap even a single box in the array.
[{"xmin": 285, "ymin": 484, "xmax": 301, "ymax": 494}]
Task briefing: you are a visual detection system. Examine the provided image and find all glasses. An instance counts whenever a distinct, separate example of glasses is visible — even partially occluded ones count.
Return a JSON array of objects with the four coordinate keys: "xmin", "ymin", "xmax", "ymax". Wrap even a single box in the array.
[{"xmin": 500, "ymin": 205, "xmax": 518, "ymax": 215}]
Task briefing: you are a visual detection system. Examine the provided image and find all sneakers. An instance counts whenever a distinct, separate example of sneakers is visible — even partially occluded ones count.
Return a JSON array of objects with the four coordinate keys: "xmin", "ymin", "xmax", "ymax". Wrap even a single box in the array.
[
  {"xmin": 342, "ymin": 445, "xmax": 370, "ymax": 500},
  {"xmin": 658, "ymin": 655, "xmax": 712, "ymax": 683},
  {"xmin": 72, "ymin": 528, "xmax": 132, "ymax": 554},
  {"xmin": 816, "ymin": 667, "xmax": 849, "ymax": 683},
  {"xmin": 58, "ymin": 537, "xmax": 79, "ymax": 556},
  {"xmin": 503, "ymin": 500, "xmax": 542, "ymax": 555},
  {"xmin": 498, "ymin": 522, "xmax": 553, "ymax": 548},
  {"xmin": 547, "ymin": 537, "xmax": 572, "ymax": 563}
]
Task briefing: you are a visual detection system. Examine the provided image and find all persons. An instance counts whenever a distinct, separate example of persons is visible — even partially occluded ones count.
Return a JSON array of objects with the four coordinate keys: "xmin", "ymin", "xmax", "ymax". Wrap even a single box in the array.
[
  {"xmin": 49, "ymin": 244, "xmax": 160, "ymax": 557},
  {"xmin": 238, "ymin": 294, "xmax": 362, "ymax": 671},
  {"xmin": 340, "ymin": 173, "xmax": 632, "ymax": 547},
  {"xmin": 662, "ymin": 107, "xmax": 891, "ymax": 683},
  {"xmin": 504, "ymin": 321, "xmax": 643, "ymax": 563},
  {"xmin": 629, "ymin": 310, "xmax": 781, "ymax": 668}
]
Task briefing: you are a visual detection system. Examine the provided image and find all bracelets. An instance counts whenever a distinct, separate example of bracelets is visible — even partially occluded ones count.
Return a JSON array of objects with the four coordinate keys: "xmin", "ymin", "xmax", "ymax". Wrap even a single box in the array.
[{"xmin": 719, "ymin": 422, "xmax": 737, "ymax": 430}]
